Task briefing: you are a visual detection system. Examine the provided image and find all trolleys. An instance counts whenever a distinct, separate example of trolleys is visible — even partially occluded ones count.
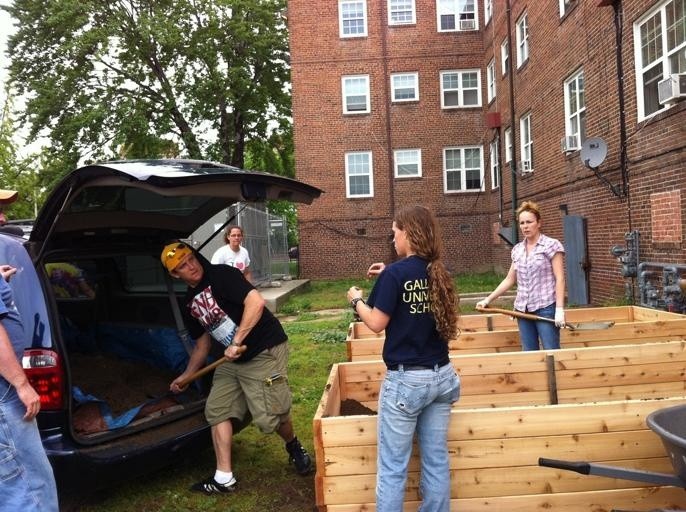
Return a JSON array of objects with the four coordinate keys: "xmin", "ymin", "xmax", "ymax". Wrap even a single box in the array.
[{"xmin": 539, "ymin": 404, "xmax": 685, "ymax": 512}]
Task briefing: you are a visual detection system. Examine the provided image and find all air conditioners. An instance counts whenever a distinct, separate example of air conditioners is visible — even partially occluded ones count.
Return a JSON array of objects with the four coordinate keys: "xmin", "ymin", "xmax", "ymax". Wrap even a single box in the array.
[{"xmin": 656, "ymin": 73, "xmax": 686, "ymax": 105}]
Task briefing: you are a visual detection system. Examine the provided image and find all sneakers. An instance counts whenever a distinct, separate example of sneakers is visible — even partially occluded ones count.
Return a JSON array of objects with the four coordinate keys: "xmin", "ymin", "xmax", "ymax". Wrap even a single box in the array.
[
  {"xmin": 190, "ymin": 476, "xmax": 238, "ymax": 496},
  {"xmin": 286, "ymin": 436, "xmax": 312, "ymax": 475}
]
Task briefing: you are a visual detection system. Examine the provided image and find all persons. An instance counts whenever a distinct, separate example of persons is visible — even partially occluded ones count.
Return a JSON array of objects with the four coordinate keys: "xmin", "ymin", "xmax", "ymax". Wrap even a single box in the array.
[
  {"xmin": 475, "ymin": 201, "xmax": 566, "ymax": 350},
  {"xmin": 346, "ymin": 206, "xmax": 460, "ymax": 511},
  {"xmin": 0, "ymin": 182, "xmax": 61, "ymax": 512},
  {"xmin": 158, "ymin": 241, "xmax": 311, "ymax": 496},
  {"xmin": 211, "ymin": 226, "xmax": 251, "ymax": 289}
]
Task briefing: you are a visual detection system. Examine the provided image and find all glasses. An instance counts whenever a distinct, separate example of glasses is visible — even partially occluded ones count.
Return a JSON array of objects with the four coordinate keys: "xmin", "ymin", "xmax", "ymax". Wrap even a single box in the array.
[
  {"xmin": 165, "ymin": 243, "xmax": 185, "ymax": 268},
  {"xmin": 231, "ymin": 234, "xmax": 242, "ymax": 237}
]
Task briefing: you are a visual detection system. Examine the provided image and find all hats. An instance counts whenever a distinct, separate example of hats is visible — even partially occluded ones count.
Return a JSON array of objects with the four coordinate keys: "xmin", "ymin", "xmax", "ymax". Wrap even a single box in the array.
[
  {"xmin": 0, "ymin": 190, "xmax": 19, "ymax": 205},
  {"xmin": 160, "ymin": 243, "xmax": 192, "ymax": 272}
]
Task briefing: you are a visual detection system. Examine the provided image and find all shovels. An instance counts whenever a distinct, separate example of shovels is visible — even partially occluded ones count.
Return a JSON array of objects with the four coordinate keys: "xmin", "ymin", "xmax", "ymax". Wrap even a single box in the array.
[
  {"xmin": 146, "ymin": 345, "xmax": 247, "ymax": 400},
  {"xmin": 475, "ymin": 305, "xmax": 615, "ymax": 331}
]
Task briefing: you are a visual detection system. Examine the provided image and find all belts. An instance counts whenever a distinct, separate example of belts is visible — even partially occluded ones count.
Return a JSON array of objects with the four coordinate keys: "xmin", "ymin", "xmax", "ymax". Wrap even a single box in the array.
[{"xmin": 387, "ymin": 357, "xmax": 449, "ymax": 371}]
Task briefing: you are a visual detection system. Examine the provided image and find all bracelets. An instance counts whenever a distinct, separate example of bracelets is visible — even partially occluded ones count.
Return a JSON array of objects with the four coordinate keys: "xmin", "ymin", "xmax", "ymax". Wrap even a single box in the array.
[
  {"xmin": 351, "ymin": 298, "xmax": 364, "ymax": 307},
  {"xmin": 230, "ymin": 344, "xmax": 241, "ymax": 347}
]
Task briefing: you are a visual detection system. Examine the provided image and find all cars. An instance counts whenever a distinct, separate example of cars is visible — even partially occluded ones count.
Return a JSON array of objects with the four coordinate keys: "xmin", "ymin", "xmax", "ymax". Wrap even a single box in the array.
[{"xmin": 0, "ymin": 158, "xmax": 324, "ymax": 470}]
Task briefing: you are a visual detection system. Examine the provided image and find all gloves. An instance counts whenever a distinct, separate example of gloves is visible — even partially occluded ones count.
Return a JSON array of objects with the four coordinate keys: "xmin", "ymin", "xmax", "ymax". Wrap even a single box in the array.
[
  {"xmin": 554, "ymin": 307, "xmax": 566, "ymax": 329},
  {"xmin": 475, "ymin": 297, "xmax": 490, "ymax": 309}
]
